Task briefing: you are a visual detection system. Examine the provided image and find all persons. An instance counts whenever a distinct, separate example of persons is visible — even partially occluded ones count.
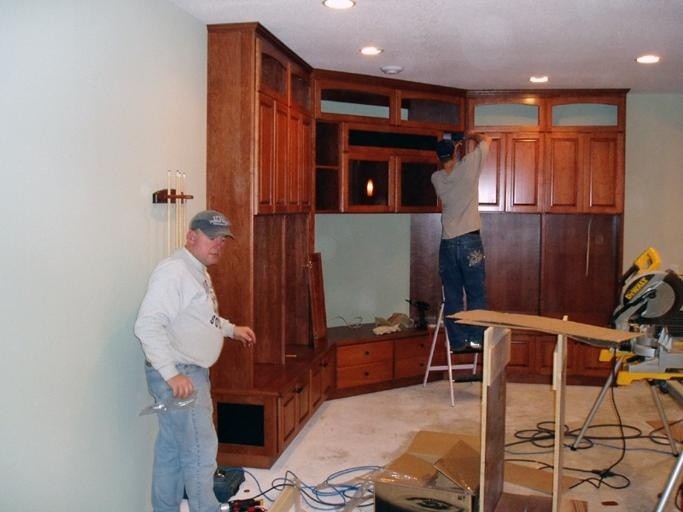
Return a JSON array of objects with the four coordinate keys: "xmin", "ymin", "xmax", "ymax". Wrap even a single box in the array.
[
  {"xmin": 430, "ymin": 128, "xmax": 493, "ymax": 353},
  {"xmin": 132, "ymin": 208, "xmax": 258, "ymax": 512}
]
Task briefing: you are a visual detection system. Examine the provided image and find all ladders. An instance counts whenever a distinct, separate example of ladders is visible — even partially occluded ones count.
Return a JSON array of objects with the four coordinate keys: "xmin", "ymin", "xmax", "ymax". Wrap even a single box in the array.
[{"xmin": 422, "ymin": 285, "xmax": 479, "ymax": 407}]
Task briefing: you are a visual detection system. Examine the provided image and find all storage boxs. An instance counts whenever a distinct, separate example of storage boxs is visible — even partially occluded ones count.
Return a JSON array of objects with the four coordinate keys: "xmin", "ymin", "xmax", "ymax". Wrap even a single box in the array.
[{"xmin": 370, "ymin": 429, "xmax": 481, "ymax": 512}]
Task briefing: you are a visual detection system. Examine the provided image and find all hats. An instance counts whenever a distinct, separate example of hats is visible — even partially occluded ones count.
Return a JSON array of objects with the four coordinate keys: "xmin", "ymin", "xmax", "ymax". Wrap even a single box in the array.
[
  {"xmin": 189, "ymin": 209, "xmax": 237, "ymax": 241},
  {"xmin": 435, "ymin": 139, "xmax": 455, "ymax": 158}
]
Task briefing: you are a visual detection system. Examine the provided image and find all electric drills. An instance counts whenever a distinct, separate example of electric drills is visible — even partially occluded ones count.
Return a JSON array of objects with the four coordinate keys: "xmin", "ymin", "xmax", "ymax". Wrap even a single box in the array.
[{"xmin": 405, "ymin": 299, "xmax": 431, "ymax": 330}]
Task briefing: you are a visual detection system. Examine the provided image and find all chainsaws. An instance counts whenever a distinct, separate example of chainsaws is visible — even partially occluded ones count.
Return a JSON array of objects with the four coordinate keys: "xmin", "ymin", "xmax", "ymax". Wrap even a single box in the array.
[{"xmin": 598, "ymin": 247, "xmax": 683, "ymax": 385}]
